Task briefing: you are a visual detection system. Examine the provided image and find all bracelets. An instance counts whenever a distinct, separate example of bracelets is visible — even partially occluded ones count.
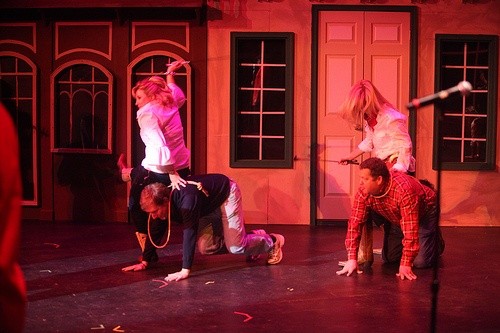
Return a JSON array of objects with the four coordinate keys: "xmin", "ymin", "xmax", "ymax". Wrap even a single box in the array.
[
  {"xmin": 140, "ymin": 262, "xmax": 148, "ymax": 268},
  {"xmin": 165, "ymin": 72, "xmax": 176, "ymax": 75}
]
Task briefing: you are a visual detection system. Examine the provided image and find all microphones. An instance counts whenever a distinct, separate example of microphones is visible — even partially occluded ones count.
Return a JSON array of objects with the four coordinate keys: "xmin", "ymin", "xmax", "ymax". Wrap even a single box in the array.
[{"xmin": 406, "ymin": 81, "xmax": 472, "ymax": 111}]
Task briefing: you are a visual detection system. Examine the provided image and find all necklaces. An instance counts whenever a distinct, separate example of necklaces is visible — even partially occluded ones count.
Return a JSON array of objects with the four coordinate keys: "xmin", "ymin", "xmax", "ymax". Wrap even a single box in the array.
[{"xmin": 372, "ymin": 172, "xmax": 392, "ymax": 199}]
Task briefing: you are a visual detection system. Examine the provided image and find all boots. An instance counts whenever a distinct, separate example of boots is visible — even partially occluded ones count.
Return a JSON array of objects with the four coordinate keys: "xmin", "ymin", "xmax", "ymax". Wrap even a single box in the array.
[
  {"xmin": 116, "ymin": 167, "xmax": 133, "ymax": 184},
  {"xmin": 136, "ymin": 231, "xmax": 159, "ymax": 262}
]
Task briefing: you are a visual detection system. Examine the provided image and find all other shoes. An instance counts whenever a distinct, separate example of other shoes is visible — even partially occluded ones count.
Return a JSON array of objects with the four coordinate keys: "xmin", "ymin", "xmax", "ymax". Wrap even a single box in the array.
[
  {"xmin": 440, "ymin": 233, "xmax": 446, "ymax": 256},
  {"xmin": 338, "ymin": 261, "xmax": 347, "ymax": 267}
]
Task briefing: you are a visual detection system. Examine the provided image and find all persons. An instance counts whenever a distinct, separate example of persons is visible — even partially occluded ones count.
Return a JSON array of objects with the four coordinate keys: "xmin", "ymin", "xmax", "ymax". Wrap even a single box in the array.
[
  {"xmin": 335, "ymin": 157, "xmax": 443, "ymax": 280},
  {"xmin": 0, "ymin": 104, "xmax": 28, "ymax": 333},
  {"xmin": 123, "ymin": 61, "xmax": 285, "ymax": 281},
  {"xmin": 337, "ymin": 81, "xmax": 416, "ymax": 268}
]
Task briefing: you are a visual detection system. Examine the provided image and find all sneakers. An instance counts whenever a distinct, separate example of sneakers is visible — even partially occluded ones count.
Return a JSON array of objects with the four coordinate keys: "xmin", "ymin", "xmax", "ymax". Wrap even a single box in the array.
[
  {"xmin": 267, "ymin": 233, "xmax": 284, "ymax": 264},
  {"xmin": 247, "ymin": 229, "xmax": 266, "ymax": 261}
]
